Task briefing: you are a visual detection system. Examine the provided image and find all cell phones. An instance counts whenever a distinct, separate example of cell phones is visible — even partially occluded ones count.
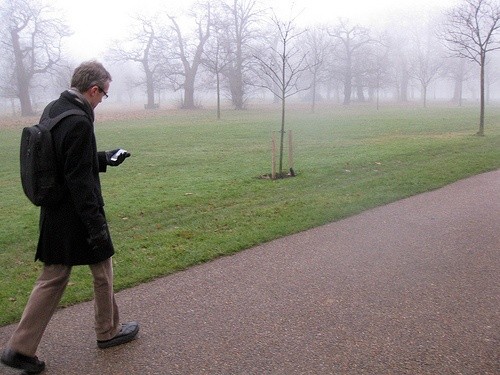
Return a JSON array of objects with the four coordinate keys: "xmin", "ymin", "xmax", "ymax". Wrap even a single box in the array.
[{"xmin": 111, "ymin": 149, "xmax": 126, "ymax": 161}]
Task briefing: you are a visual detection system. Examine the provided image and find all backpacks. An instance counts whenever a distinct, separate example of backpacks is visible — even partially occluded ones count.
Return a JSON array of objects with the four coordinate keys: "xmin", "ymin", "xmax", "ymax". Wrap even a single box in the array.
[{"xmin": 20, "ymin": 99, "xmax": 90, "ymax": 207}]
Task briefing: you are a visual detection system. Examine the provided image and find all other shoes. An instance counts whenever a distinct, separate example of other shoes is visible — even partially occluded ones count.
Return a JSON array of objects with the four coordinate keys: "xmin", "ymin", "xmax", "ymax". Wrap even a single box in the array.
[
  {"xmin": 97, "ymin": 321, "xmax": 140, "ymax": 349},
  {"xmin": 0, "ymin": 349, "xmax": 45, "ymax": 373}
]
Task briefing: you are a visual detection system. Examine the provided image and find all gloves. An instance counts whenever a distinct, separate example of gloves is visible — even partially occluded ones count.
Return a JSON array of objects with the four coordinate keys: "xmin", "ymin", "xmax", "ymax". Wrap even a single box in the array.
[{"xmin": 105, "ymin": 149, "xmax": 131, "ymax": 166}]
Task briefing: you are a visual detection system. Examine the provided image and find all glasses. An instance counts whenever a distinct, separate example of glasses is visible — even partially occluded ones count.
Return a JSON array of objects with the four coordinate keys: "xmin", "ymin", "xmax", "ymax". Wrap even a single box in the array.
[{"xmin": 98, "ymin": 86, "xmax": 108, "ymax": 99}]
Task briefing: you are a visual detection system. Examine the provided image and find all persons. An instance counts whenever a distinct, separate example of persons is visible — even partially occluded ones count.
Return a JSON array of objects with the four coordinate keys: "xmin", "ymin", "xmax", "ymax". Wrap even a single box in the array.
[{"xmin": 0, "ymin": 61, "xmax": 139, "ymax": 373}]
